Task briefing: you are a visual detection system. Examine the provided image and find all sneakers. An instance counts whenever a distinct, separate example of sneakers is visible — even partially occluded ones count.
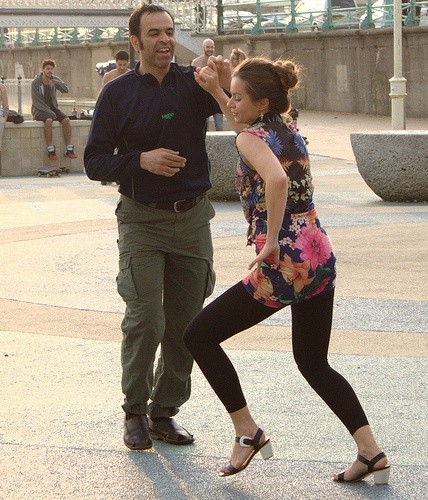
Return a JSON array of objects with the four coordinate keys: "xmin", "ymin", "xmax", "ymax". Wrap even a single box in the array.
[
  {"xmin": 64, "ymin": 144, "xmax": 77, "ymax": 159},
  {"xmin": 47, "ymin": 145, "xmax": 58, "ymax": 160}
]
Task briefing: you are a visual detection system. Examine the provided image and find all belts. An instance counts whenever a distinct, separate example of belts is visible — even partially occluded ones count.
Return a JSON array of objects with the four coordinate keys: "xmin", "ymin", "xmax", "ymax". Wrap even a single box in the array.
[{"xmin": 144, "ymin": 193, "xmax": 206, "ymax": 213}]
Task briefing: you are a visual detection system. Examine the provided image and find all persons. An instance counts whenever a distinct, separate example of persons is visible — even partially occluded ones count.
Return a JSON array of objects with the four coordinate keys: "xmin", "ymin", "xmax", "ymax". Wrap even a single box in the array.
[
  {"xmin": 191, "ymin": 38, "xmax": 224, "ymax": 132},
  {"xmin": 0, "ymin": 83, "xmax": 9, "ymax": 152},
  {"xmin": 230, "ymin": 48, "xmax": 246, "ymax": 68},
  {"xmin": 100, "ymin": 51, "xmax": 130, "ymax": 185},
  {"xmin": 84, "ymin": 4, "xmax": 233, "ymax": 450},
  {"xmin": 183, "ymin": 57, "xmax": 390, "ymax": 485},
  {"xmin": 31, "ymin": 59, "xmax": 77, "ymax": 160}
]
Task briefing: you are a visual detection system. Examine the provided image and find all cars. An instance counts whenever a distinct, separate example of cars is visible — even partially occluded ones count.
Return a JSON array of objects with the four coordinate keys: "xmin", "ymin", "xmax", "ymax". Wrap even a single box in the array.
[{"xmin": 174, "ymin": 0, "xmax": 428, "ymax": 32}]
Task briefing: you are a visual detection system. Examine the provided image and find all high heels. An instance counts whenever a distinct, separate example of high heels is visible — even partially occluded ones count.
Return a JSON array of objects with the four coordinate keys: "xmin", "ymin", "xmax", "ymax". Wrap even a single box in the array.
[
  {"xmin": 333, "ymin": 453, "xmax": 391, "ymax": 485},
  {"xmin": 218, "ymin": 428, "xmax": 273, "ymax": 477}
]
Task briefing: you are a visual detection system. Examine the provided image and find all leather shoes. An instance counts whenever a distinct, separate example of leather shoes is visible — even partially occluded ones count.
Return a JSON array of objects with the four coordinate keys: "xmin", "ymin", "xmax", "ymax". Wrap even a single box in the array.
[
  {"xmin": 125, "ymin": 412, "xmax": 153, "ymax": 449},
  {"xmin": 146, "ymin": 416, "xmax": 195, "ymax": 444}
]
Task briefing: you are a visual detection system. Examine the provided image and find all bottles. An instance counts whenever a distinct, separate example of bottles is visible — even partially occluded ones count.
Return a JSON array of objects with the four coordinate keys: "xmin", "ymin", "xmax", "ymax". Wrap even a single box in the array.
[{"xmin": 71, "ymin": 106, "xmax": 77, "ymax": 117}]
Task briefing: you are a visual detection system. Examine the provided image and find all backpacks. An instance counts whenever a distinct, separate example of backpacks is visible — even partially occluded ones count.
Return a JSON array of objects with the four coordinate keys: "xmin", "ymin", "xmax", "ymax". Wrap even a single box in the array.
[{"xmin": 7, "ymin": 110, "xmax": 24, "ymax": 124}]
